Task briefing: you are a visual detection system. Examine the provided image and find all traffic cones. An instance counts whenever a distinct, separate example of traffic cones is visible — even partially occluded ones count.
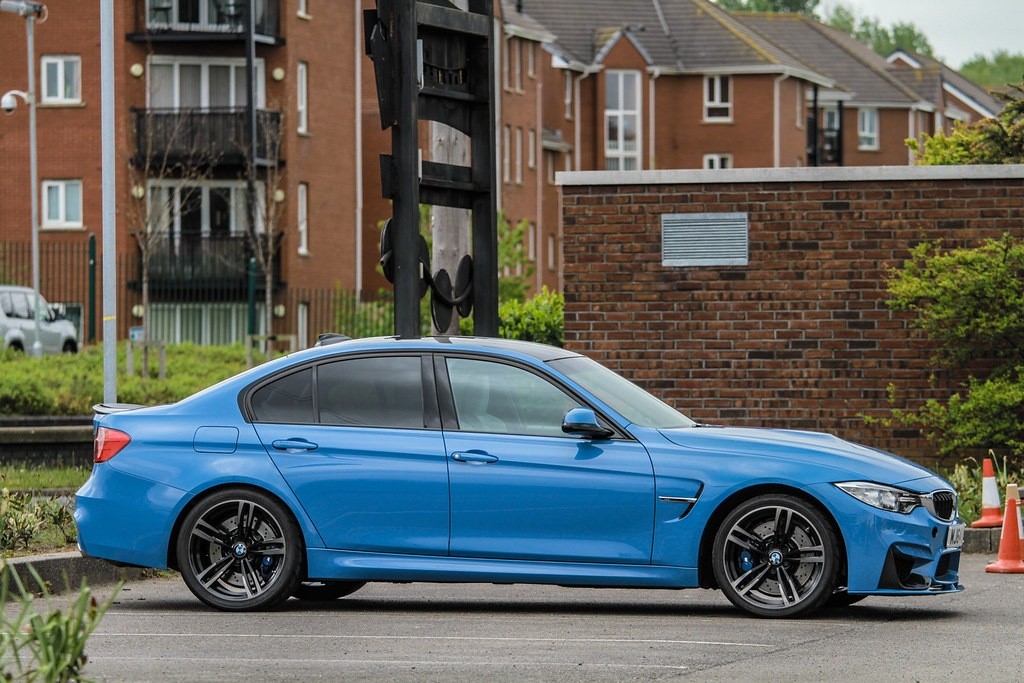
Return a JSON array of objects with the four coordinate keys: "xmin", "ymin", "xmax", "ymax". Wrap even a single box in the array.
[
  {"xmin": 984, "ymin": 483, "xmax": 1023, "ymax": 574},
  {"xmin": 970, "ymin": 458, "xmax": 1004, "ymax": 528}
]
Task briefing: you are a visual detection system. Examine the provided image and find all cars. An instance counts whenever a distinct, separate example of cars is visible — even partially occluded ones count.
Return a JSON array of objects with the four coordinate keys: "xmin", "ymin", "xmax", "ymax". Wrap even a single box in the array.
[
  {"xmin": 0, "ymin": 284, "xmax": 79, "ymax": 358},
  {"xmin": 70, "ymin": 332, "xmax": 966, "ymax": 620}
]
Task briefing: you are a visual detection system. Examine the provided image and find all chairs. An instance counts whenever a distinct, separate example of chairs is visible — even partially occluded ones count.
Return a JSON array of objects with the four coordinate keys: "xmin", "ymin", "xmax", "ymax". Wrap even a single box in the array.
[{"xmin": 455, "ymin": 373, "xmax": 509, "ymax": 433}]
